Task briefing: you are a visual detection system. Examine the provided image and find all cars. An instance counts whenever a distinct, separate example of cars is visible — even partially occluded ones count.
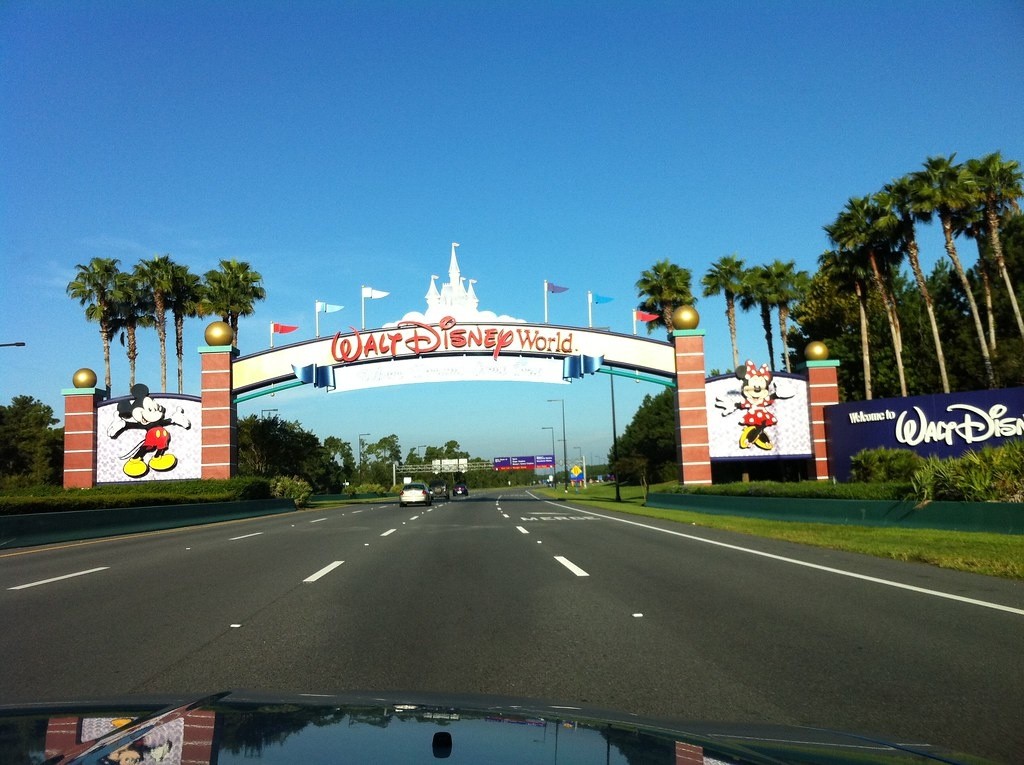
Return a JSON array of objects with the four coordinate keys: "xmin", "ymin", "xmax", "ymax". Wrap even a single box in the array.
[
  {"xmin": 453, "ymin": 483, "xmax": 469, "ymax": 497},
  {"xmin": 427, "ymin": 480, "xmax": 449, "ymax": 501},
  {"xmin": 399, "ymin": 483, "xmax": 434, "ymax": 507}
]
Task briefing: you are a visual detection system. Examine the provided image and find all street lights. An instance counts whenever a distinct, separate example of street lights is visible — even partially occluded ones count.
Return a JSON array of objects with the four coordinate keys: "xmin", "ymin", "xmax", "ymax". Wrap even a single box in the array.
[
  {"xmin": 548, "ymin": 399, "xmax": 568, "ymax": 491},
  {"xmin": 542, "ymin": 427, "xmax": 557, "ymax": 489}
]
